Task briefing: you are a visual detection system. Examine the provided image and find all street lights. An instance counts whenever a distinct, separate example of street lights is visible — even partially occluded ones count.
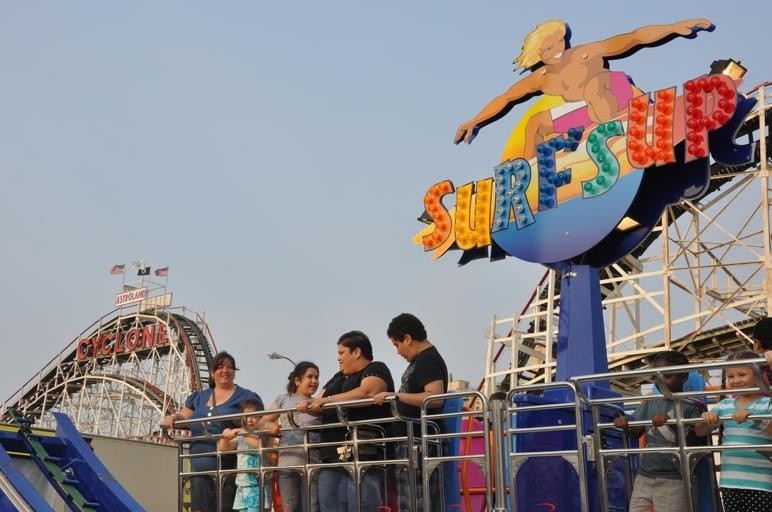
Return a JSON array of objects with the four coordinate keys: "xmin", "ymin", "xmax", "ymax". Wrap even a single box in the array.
[{"xmin": 268, "ymin": 351, "xmax": 295, "ymax": 367}]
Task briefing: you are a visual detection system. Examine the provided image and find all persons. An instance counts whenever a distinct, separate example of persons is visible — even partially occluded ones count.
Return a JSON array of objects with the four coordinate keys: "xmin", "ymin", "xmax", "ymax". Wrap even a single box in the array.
[
  {"xmin": 255, "ymin": 362, "xmax": 319, "ymax": 512},
  {"xmin": 373, "ymin": 313, "xmax": 448, "ymax": 511},
  {"xmin": 455, "ymin": 19, "xmax": 714, "ymax": 161},
  {"xmin": 295, "ymin": 330, "xmax": 394, "ymax": 512},
  {"xmin": 220, "ymin": 399, "xmax": 276, "ymax": 512},
  {"xmin": 613, "ymin": 317, "xmax": 772, "ymax": 512},
  {"xmin": 165, "ymin": 352, "xmax": 264, "ymax": 512}
]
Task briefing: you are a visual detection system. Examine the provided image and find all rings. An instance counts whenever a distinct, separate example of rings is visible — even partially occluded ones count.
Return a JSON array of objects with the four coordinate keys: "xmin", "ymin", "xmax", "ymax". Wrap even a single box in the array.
[{"xmin": 297, "ymin": 405, "xmax": 300, "ymax": 408}]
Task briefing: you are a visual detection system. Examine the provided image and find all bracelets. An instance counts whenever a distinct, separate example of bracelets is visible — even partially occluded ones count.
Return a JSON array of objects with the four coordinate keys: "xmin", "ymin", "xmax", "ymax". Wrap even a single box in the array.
[{"xmin": 328, "ymin": 396, "xmax": 331, "ymax": 403}]
